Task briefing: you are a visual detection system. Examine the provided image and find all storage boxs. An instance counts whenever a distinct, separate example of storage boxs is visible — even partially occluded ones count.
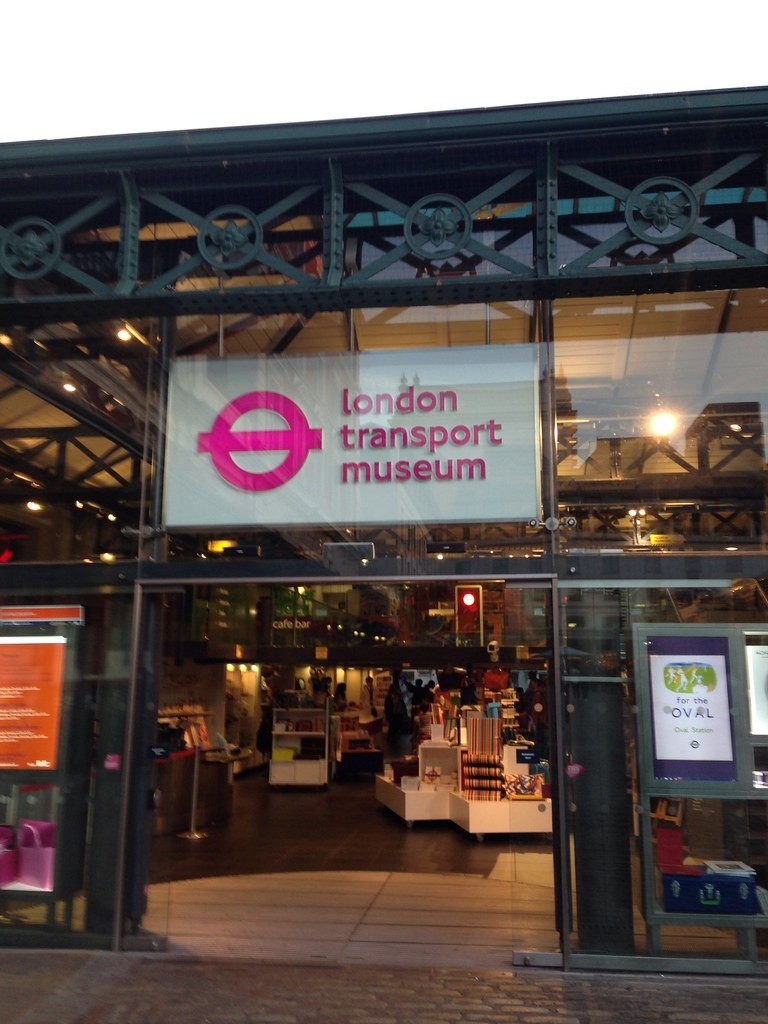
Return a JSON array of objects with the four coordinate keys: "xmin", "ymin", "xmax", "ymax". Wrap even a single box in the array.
[{"xmin": 663, "ymin": 863, "xmax": 757, "ymax": 916}]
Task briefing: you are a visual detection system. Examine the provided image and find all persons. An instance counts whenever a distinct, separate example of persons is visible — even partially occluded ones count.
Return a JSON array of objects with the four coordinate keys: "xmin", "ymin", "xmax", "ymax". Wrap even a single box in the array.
[
  {"xmin": 360, "ymin": 676, "xmax": 378, "ymax": 708},
  {"xmin": 312, "ymin": 677, "xmax": 347, "ymax": 712},
  {"xmin": 517, "ymin": 671, "xmax": 547, "ymax": 745},
  {"xmin": 384, "ymin": 671, "xmax": 435, "ymax": 733}
]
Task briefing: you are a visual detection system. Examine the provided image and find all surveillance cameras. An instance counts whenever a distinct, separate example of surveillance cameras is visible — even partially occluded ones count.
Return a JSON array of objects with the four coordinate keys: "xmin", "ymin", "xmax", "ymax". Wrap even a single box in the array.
[{"xmin": 488, "ymin": 642, "xmax": 500, "ymax": 654}]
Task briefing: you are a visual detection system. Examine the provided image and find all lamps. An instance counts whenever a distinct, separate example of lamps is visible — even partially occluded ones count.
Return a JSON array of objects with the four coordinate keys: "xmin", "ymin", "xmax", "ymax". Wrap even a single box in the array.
[{"xmin": 487, "ymin": 641, "xmax": 500, "ymax": 663}]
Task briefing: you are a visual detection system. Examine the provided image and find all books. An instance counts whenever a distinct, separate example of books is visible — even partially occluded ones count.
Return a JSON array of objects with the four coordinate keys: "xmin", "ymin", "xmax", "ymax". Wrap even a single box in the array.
[
  {"xmin": 703, "ymin": 860, "xmax": 757, "ymax": 878},
  {"xmin": 756, "ymin": 886, "xmax": 768, "ymax": 915}
]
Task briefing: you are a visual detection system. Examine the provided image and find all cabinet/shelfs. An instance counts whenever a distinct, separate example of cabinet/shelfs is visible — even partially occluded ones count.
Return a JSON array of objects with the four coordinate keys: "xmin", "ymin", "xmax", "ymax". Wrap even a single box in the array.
[
  {"xmin": 269, "ymin": 698, "xmax": 330, "ymax": 788},
  {"xmin": 376, "ymin": 699, "xmax": 553, "ymax": 842}
]
